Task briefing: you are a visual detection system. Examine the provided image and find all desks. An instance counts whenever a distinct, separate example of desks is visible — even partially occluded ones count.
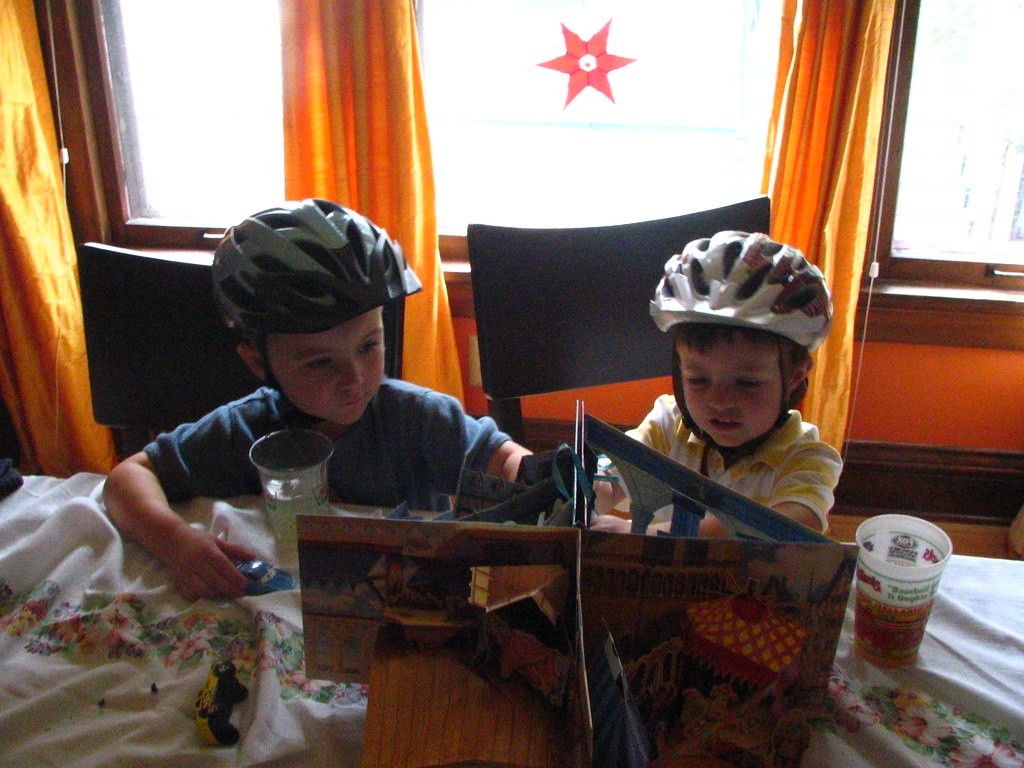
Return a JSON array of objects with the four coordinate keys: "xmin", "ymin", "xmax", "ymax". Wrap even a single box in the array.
[{"xmin": 1, "ymin": 495, "xmax": 1023, "ymax": 768}]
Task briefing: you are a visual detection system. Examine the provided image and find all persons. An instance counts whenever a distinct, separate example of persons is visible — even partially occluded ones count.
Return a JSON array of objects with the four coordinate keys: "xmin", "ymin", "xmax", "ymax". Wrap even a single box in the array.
[
  {"xmin": 592, "ymin": 231, "xmax": 843, "ymax": 537},
  {"xmin": 104, "ymin": 199, "xmax": 534, "ymax": 600}
]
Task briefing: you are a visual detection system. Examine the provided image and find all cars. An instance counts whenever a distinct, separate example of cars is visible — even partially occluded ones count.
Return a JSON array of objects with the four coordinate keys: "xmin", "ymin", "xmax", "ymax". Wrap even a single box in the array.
[{"xmin": 229, "ymin": 558, "xmax": 296, "ymax": 595}]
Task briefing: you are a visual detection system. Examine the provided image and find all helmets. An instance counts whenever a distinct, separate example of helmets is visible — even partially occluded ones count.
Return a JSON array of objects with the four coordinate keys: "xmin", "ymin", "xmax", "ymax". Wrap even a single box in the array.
[
  {"xmin": 210, "ymin": 197, "xmax": 423, "ymax": 330},
  {"xmin": 649, "ymin": 230, "xmax": 835, "ymax": 352}
]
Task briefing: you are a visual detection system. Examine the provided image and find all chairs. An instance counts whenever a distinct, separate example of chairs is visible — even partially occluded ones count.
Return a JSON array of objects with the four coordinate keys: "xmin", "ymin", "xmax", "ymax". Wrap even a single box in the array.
[
  {"xmin": 466, "ymin": 192, "xmax": 770, "ymax": 448},
  {"xmin": 76, "ymin": 240, "xmax": 407, "ymax": 461}
]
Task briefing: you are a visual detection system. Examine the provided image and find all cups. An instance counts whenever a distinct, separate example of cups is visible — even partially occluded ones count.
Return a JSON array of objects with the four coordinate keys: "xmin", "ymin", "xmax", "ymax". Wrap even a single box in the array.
[
  {"xmin": 251, "ymin": 429, "xmax": 336, "ymax": 540},
  {"xmin": 854, "ymin": 514, "xmax": 953, "ymax": 666}
]
